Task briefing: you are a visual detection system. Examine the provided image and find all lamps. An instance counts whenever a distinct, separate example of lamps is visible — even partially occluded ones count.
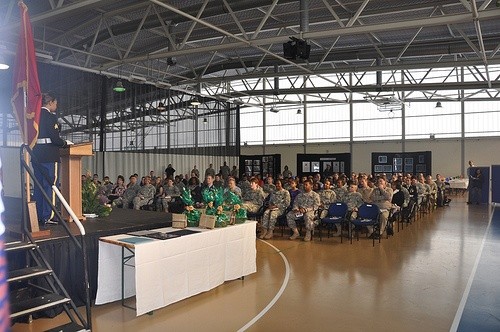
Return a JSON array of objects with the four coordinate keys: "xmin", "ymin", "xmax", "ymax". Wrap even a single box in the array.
[
  {"xmin": 190, "ymin": 96, "xmax": 201, "ymax": 105},
  {"xmin": 113, "ymin": 63, "xmax": 125, "ymax": 91},
  {"xmin": 157, "ymin": 102, "xmax": 166, "ymax": 110}
]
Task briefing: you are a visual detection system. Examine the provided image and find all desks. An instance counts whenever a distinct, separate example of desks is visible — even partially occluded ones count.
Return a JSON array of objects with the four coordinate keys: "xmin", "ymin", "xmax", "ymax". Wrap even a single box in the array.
[
  {"xmin": 436, "ymin": 178, "xmax": 469, "ymax": 196},
  {"xmin": 100, "ymin": 220, "xmax": 258, "ymax": 315}
]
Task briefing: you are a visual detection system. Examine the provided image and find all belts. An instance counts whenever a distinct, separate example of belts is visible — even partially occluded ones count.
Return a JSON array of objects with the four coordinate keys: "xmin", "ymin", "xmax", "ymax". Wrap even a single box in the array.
[{"xmin": 36, "ymin": 138, "xmax": 52, "ymax": 144}]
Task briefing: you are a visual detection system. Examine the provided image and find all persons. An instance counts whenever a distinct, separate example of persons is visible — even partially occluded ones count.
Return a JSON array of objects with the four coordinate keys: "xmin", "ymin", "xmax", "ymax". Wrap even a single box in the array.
[
  {"xmin": 224, "ymin": 180, "xmax": 241, "ymax": 204},
  {"xmin": 156, "ymin": 179, "xmax": 181, "ymax": 213},
  {"xmin": 260, "ymin": 179, "xmax": 291, "ymax": 240},
  {"xmin": 230, "ymin": 166, "xmax": 239, "ymax": 179},
  {"xmin": 240, "ymin": 177, "xmax": 266, "ymax": 215},
  {"xmin": 323, "ymin": 165, "xmax": 333, "ymax": 177},
  {"xmin": 31, "ymin": 92, "xmax": 73, "ymax": 226},
  {"xmin": 467, "ymin": 170, "xmax": 482, "ymax": 205},
  {"xmin": 164, "ymin": 164, "xmax": 175, "ymax": 180},
  {"xmin": 82, "ymin": 171, "xmax": 138, "ymax": 208},
  {"xmin": 112, "ymin": 177, "xmax": 140, "ymax": 209},
  {"xmin": 150, "ymin": 170, "xmax": 445, "ymax": 239},
  {"xmin": 221, "ymin": 161, "xmax": 230, "ymax": 180},
  {"xmin": 287, "ymin": 180, "xmax": 320, "ymax": 240},
  {"xmin": 191, "ymin": 165, "xmax": 200, "ymax": 178},
  {"xmin": 283, "ymin": 165, "xmax": 292, "ymax": 179},
  {"xmin": 468, "ymin": 161, "xmax": 475, "ymax": 167},
  {"xmin": 205, "ymin": 164, "xmax": 215, "ymax": 179},
  {"xmin": 194, "ymin": 175, "xmax": 218, "ymax": 208},
  {"xmin": 133, "ymin": 175, "xmax": 155, "ymax": 211}
]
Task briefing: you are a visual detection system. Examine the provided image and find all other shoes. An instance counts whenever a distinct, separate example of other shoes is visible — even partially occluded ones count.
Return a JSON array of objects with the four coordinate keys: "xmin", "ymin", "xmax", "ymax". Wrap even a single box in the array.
[
  {"xmin": 374, "ymin": 234, "xmax": 383, "ymax": 239},
  {"xmin": 288, "ymin": 231, "xmax": 300, "ymax": 240},
  {"xmin": 332, "ymin": 231, "xmax": 342, "ymax": 237},
  {"xmin": 45, "ymin": 220, "xmax": 59, "ymax": 225},
  {"xmin": 259, "ymin": 229, "xmax": 274, "ymax": 240},
  {"xmin": 303, "ymin": 231, "xmax": 311, "ymax": 242}
]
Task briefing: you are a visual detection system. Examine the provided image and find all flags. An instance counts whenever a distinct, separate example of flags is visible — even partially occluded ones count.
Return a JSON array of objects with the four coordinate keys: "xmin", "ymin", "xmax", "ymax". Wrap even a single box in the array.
[{"xmin": 13, "ymin": 2, "xmax": 42, "ymax": 151}]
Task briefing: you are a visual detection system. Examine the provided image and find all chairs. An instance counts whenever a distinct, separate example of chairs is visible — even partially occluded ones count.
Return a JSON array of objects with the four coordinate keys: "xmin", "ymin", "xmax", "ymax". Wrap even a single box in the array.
[{"xmin": 317, "ymin": 192, "xmax": 435, "ymax": 247}]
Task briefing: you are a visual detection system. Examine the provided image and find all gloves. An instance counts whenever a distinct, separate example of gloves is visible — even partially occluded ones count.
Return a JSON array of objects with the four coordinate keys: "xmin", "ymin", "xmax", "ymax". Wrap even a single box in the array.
[{"xmin": 65, "ymin": 139, "xmax": 75, "ymax": 146}]
